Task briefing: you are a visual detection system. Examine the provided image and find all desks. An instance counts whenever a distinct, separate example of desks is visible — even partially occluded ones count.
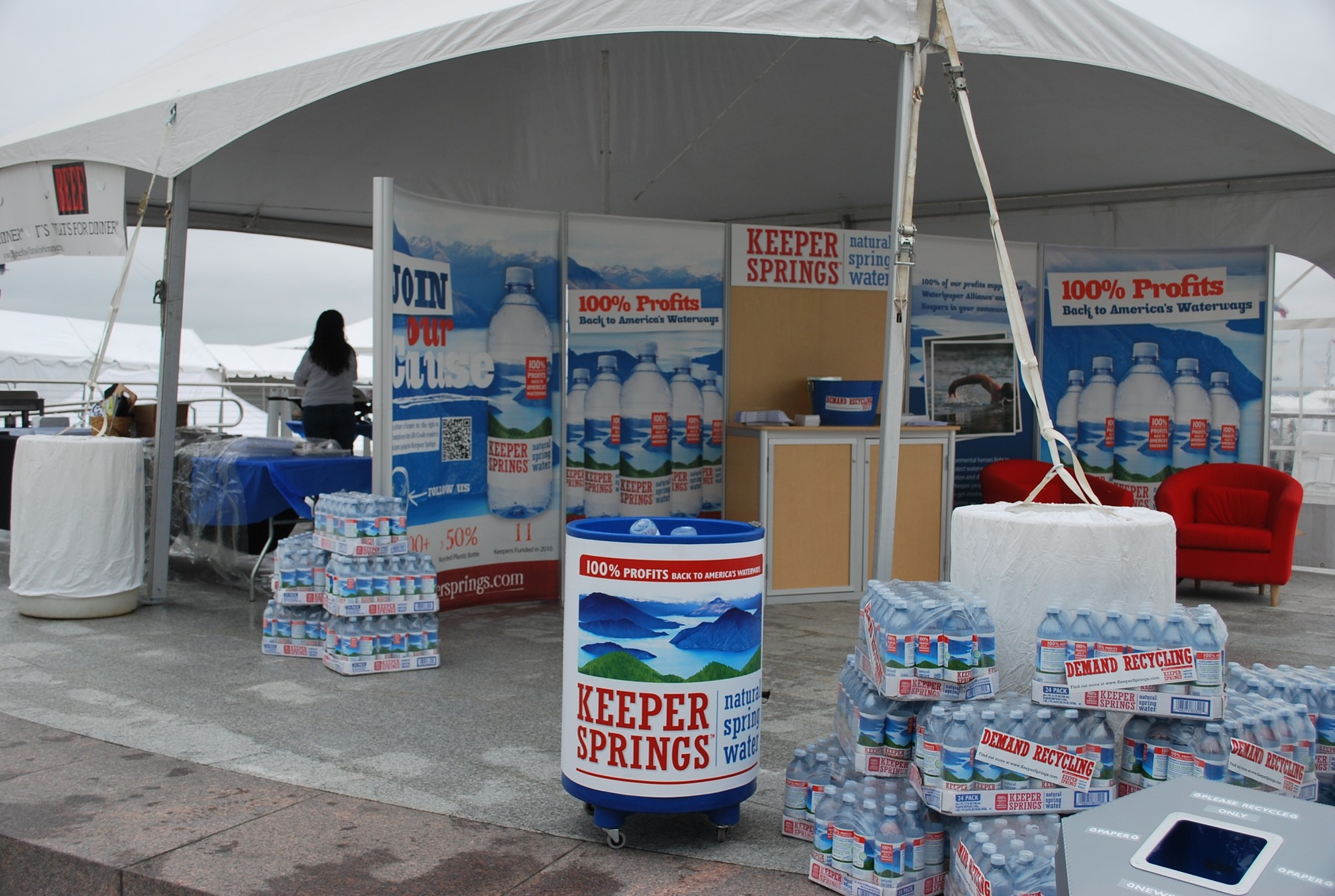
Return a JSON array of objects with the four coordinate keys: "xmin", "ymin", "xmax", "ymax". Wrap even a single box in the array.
[
  {"xmin": 184, "ymin": 454, "xmax": 374, "ymax": 607},
  {"xmin": 1057, "ymin": 771, "xmax": 1335, "ymax": 896},
  {"xmin": 1265, "ymin": 397, "xmax": 1334, "ymax": 431}
]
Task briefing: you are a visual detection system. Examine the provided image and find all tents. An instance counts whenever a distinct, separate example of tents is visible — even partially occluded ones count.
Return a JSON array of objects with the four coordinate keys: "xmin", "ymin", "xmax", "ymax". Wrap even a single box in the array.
[
  {"xmin": 0, "ymin": 0, "xmax": 1335, "ymax": 605},
  {"xmin": 0, "ymin": 309, "xmax": 220, "ymax": 432},
  {"xmin": 265, "ymin": 317, "xmax": 374, "ymax": 354},
  {"xmin": 202, "ymin": 342, "xmax": 373, "ymax": 447}
]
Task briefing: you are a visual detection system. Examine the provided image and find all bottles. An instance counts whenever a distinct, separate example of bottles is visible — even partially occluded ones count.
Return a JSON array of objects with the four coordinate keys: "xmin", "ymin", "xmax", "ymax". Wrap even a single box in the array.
[
  {"xmin": 1119, "ymin": 661, "xmax": 1335, "ymax": 808},
  {"xmin": 584, "ymin": 356, "xmax": 623, "ymax": 517},
  {"xmin": 629, "ymin": 518, "xmax": 661, "ymax": 536},
  {"xmin": 670, "ymin": 526, "xmax": 697, "ymax": 536},
  {"xmin": 1078, "ymin": 357, "xmax": 1117, "ymax": 483},
  {"xmin": 855, "ymin": 579, "xmax": 994, "ymax": 702},
  {"xmin": 261, "ymin": 599, "xmax": 331, "ymax": 659},
  {"xmin": 1209, "ymin": 371, "xmax": 1240, "ymax": 464},
  {"xmin": 620, "ymin": 341, "xmax": 673, "ymax": 517},
  {"xmin": 669, "ymin": 357, "xmax": 704, "ymax": 516},
  {"xmin": 1170, "ymin": 358, "xmax": 1212, "ymax": 475},
  {"xmin": 487, "ymin": 266, "xmax": 552, "ymax": 518},
  {"xmin": 915, "ymin": 691, "xmax": 1115, "ymax": 816},
  {"xmin": 1056, "ymin": 370, "xmax": 1084, "ymax": 468},
  {"xmin": 322, "ymin": 610, "xmax": 438, "ymax": 675},
  {"xmin": 312, "ymin": 491, "xmax": 407, "ymax": 557},
  {"xmin": 270, "ymin": 531, "xmax": 332, "ymax": 605},
  {"xmin": 566, "ymin": 368, "xmax": 592, "ymax": 515},
  {"xmin": 322, "ymin": 551, "xmax": 437, "ymax": 616},
  {"xmin": 1033, "ymin": 603, "xmax": 1225, "ymax": 721},
  {"xmin": 833, "ymin": 653, "xmax": 922, "ymax": 777},
  {"xmin": 700, "ymin": 371, "xmax": 723, "ymax": 512},
  {"xmin": 1114, "ymin": 342, "xmax": 1175, "ymax": 510},
  {"xmin": 781, "ymin": 733, "xmax": 1061, "ymax": 896}
]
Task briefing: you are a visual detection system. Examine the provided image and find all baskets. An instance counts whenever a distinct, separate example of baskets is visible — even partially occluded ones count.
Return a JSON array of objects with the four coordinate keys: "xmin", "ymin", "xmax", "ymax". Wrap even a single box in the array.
[{"xmin": 90, "ymin": 417, "xmax": 132, "ymax": 438}]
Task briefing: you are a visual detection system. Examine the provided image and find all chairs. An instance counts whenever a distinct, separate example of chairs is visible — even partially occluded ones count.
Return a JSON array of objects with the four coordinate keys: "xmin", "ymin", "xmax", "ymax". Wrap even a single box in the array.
[{"xmin": 1285, "ymin": 430, "xmax": 1335, "ymax": 580}]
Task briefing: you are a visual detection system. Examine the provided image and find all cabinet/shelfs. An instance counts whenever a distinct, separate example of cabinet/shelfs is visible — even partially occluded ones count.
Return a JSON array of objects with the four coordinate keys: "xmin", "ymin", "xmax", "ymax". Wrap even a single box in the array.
[{"xmin": 718, "ymin": 419, "xmax": 962, "ymax": 610}]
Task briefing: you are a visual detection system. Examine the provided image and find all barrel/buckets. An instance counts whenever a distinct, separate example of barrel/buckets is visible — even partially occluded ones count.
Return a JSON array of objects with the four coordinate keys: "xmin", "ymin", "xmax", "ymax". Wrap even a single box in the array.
[
  {"xmin": 807, "ymin": 379, "xmax": 882, "ymax": 426},
  {"xmin": 561, "ymin": 517, "xmax": 765, "ymax": 829}
]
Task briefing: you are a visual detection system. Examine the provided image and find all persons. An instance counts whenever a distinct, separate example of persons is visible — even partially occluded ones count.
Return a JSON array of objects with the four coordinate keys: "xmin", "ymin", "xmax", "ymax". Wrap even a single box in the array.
[
  {"xmin": 948, "ymin": 374, "xmax": 1014, "ymax": 406},
  {"xmin": 292, "ymin": 310, "xmax": 357, "ymax": 455}
]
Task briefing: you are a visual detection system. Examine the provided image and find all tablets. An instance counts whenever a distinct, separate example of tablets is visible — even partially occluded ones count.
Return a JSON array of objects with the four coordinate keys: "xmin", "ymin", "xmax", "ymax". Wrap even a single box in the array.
[{"xmin": 1129, "ymin": 811, "xmax": 1286, "ymax": 895}]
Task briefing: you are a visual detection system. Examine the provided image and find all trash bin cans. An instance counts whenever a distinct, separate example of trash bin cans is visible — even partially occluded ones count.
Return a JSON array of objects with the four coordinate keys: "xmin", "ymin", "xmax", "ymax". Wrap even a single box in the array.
[
  {"xmin": 564, "ymin": 515, "xmax": 765, "ymax": 848},
  {"xmin": 12, "ymin": 436, "xmax": 145, "ymax": 620}
]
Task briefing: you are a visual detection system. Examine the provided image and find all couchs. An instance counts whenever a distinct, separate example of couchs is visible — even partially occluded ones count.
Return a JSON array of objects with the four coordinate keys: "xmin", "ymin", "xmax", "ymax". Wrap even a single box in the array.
[
  {"xmin": 1152, "ymin": 461, "xmax": 1305, "ymax": 608},
  {"xmin": 978, "ymin": 456, "xmax": 1136, "ymax": 507}
]
